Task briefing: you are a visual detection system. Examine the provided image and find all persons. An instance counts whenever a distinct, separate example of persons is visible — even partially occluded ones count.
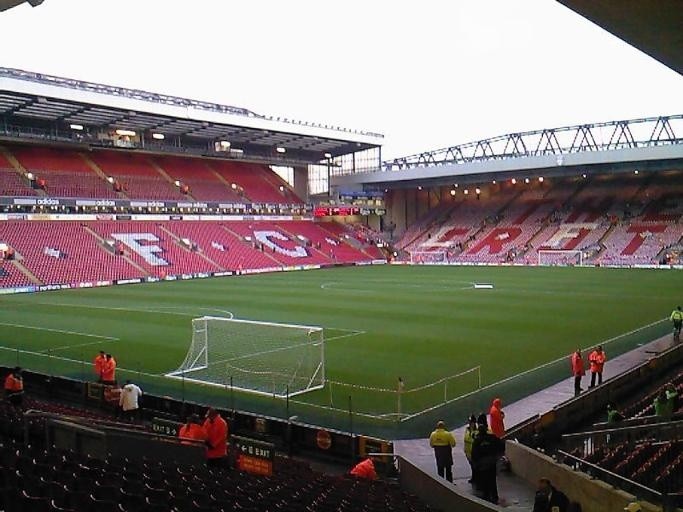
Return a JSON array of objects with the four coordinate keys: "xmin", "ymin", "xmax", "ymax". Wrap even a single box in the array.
[
  {"xmin": 103, "ymin": 379, "xmax": 123, "ymax": 420},
  {"xmin": 529, "ymin": 476, "xmax": 570, "ymax": 512},
  {"xmin": 663, "ymin": 381, "xmax": 679, "ymax": 413},
  {"xmin": 622, "ymin": 503, "xmax": 641, "ymax": 512},
  {"xmin": 585, "ymin": 345, "xmax": 607, "ymax": 389},
  {"xmin": 599, "ymin": 399, "xmax": 635, "ymax": 451},
  {"xmin": 198, "ymin": 405, "xmax": 228, "ymax": 460},
  {"xmin": 489, "ymin": 398, "xmax": 505, "ymax": 438},
  {"xmin": 470, "ymin": 412, "xmax": 508, "ymax": 504},
  {"xmin": 429, "ymin": 421, "xmax": 456, "ymax": 484},
  {"xmin": 94, "ymin": 350, "xmax": 107, "ymax": 376},
  {"xmin": 426, "ymin": 201, "xmax": 677, "ymax": 265},
  {"xmin": 462, "ymin": 413, "xmax": 478, "ymax": 483},
  {"xmin": 177, "ymin": 412, "xmax": 203, "ymax": 448},
  {"xmin": 570, "ymin": 347, "xmax": 585, "ymax": 398},
  {"xmin": 4, "ymin": 365, "xmax": 25, "ymax": 407},
  {"xmin": 652, "ymin": 389, "xmax": 671, "ymax": 443},
  {"xmin": 668, "ymin": 304, "xmax": 682, "ymax": 337},
  {"xmin": 117, "ymin": 379, "xmax": 142, "ymax": 420},
  {"xmin": 347, "ymin": 456, "xmax": 389, "ymax": 482},
  {"xmin": 102, "ymin": 353, "xmax": 117, "ymax": 384}
]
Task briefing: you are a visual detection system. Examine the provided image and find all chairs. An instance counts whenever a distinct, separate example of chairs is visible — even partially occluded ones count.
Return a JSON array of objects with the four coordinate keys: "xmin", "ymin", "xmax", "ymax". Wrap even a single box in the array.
[
  {"xmin": 563, "ymin": 372, "xmax": 683, "ymax": 492},
  {"xmin": 0, "ymin": 387, "xmax": 441, "ymax": 512}
]
